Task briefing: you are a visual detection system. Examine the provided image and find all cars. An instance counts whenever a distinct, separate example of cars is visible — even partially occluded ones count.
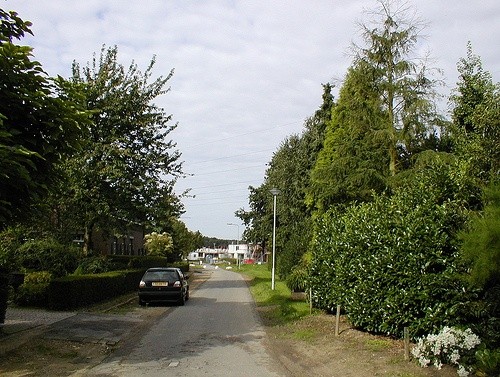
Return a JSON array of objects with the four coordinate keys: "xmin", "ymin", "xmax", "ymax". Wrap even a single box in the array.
[{"xmin": 138, "ymin": 267, "xmax": 190, "ymax": 307}]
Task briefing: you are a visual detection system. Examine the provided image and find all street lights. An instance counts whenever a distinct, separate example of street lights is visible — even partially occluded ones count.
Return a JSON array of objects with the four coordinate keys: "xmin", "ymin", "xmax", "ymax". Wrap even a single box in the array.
[
  {"xmin": 227, "ymin": 222, "xmax": 245, "ymax": 267},
  {"xmin": 269, "ymin": 187, "xmax": 280, "ymax": 291}
]
